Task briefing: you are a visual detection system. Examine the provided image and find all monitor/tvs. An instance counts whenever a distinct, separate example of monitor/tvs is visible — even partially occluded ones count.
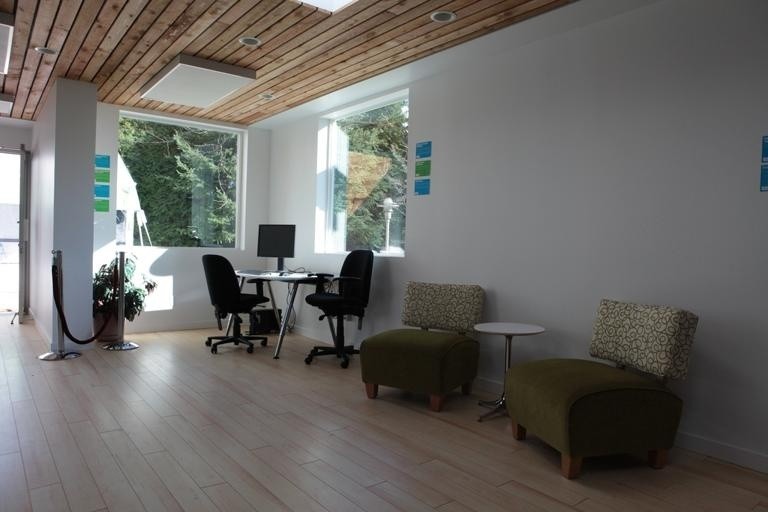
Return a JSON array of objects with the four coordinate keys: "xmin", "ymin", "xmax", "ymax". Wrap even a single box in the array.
[{"xmin": 257, "ymin": 225, "xmax": 295, "ymax": 272}]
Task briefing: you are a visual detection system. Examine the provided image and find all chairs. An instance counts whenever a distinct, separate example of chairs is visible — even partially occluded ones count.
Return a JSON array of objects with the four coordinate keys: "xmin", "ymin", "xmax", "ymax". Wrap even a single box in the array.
[
  {"xmin": 359, "ymin": 280, "xmax": 487, "ymax": 412},
  {"xmin": 200, "ymin": 251, "xmax": 374, "ymax": 368},
  {"xmin": 504, "ymin": 297, "xmax": 698, "ymax": 480}
]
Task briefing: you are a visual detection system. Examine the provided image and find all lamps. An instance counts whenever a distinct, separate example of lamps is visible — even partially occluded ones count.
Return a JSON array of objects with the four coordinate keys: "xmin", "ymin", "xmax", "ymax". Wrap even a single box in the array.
[{"xmin": 140, "ymin": 54, "xmax": 256, "ymax": 111}]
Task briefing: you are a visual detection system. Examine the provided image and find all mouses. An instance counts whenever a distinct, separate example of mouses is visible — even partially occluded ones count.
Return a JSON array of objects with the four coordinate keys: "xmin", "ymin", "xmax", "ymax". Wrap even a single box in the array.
[{"xmin": 280, "ymin": 272, "xmax": 289, "ymax": 276}]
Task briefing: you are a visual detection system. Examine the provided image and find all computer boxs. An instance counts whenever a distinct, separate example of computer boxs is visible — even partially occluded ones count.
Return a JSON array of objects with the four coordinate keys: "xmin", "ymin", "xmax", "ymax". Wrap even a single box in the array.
[{"xmin": 245, "ymin": 309, "xmax": 281, "ymax": 335}]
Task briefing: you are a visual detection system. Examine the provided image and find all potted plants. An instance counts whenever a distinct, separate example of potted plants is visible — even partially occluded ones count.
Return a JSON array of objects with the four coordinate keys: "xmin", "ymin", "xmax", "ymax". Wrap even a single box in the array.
[{"xmin": 94, "ymin": 254, "xmax": 156, "ymax": 341}]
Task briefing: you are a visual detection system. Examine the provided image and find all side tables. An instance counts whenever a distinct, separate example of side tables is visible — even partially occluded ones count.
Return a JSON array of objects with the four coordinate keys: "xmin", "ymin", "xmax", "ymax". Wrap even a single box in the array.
[{"xmin": 474, "ymin": 322, "xmax": 544, "ymax": 423}]
